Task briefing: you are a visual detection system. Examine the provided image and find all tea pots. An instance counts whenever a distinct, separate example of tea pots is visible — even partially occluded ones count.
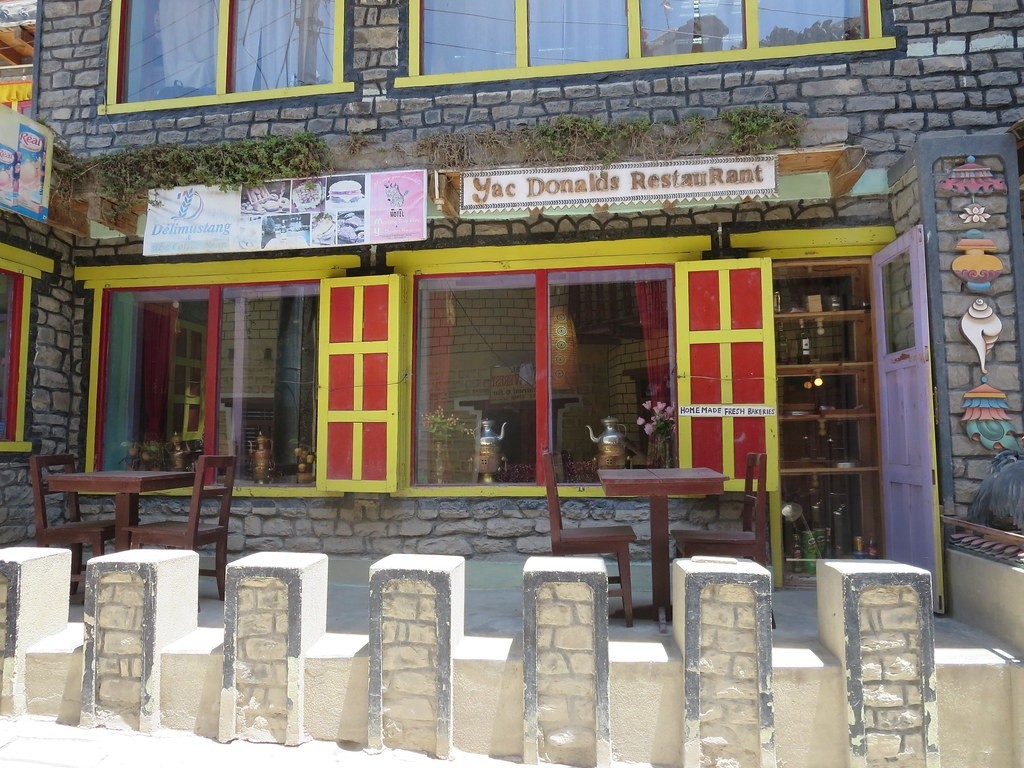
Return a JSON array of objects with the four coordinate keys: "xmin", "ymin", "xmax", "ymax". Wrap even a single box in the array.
[
  {"xmin": 585, "ymin": 416, "xmax": 627, "ymax": 453},
  {"xmin": 472, "ymin": 418, "xmax": 507, "ymax": 447}
]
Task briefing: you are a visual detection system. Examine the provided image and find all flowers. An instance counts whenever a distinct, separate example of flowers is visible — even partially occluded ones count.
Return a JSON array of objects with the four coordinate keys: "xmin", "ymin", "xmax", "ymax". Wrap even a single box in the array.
[
  {"xmin": 289, "ymin": 437, "xmax": 317, "ymax": 472},
  {"xmin": 420, "ymin": 404, "xmax": 476, "ymax": 441},
  {"xmin": 118, "ymin": 439, "xmax": 162, "ymax": 471},
  {"xmin": 636, "ymin": 399, "xmax": 677, "ymax": 436}
]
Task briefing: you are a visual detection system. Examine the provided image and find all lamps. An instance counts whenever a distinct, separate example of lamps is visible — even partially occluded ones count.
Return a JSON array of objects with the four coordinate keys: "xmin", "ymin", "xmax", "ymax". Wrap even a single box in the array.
[
  {"xmin": 813, "ymin": 316, "xmax": 826, "ymax": 335},
  {"xmin": 550, "ymin": 286, "xmax": 579, "ymax": 389}
]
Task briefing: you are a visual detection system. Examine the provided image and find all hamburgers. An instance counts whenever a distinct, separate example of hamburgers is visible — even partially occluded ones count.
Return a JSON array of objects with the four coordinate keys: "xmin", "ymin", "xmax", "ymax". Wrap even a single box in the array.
[
  {"xmin": 328, "ymin": 180, "xmax": 362, "ymax": 208},
  {"xmin": 315, "ymin": 219, "xmax": 334, "ymax": 239}
]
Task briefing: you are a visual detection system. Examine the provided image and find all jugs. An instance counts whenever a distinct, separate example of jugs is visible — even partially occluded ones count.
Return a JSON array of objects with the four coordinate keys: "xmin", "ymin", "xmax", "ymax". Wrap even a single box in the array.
[
  {"xmin": 248, "ymin": 431, "xmax": 274, "ymax": 485},
  {"xmin": 164, "ymin": 431, "xmax": 191, "ymax": 472}
]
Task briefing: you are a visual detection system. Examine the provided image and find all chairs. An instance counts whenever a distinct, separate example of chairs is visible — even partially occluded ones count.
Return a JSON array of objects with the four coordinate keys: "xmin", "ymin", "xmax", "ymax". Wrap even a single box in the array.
[
  {"xmin": 30, "ymin": 453, "xmax": 143, "ymax": 596},
  {"xmin": 123, "ymin": 453, "xmax": 237, "ymax": 613},
  {"xmin": 671, "ymin": 453, "xmax": 777, "ymax": 629},
  {"xmin": 542, "ymin": 451, "xmax": 638, "ymax": 627}
]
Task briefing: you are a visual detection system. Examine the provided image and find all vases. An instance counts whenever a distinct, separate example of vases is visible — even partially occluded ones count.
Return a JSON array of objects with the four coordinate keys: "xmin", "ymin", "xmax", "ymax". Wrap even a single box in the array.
[
  {"xmin": 435, "ymin": 442, "xmax": 453, "ymax": 485},
  {"xmin": 653, "ymin": 432, "xmax": 673, "ymax": 469},
  {"xmin": 297, "ymin": 473, "xmax": 312, "ymax": 484}
]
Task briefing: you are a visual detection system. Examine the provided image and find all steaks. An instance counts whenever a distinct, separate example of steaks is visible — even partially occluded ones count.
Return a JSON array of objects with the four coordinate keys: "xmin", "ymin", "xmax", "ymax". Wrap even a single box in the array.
[{"xmin": 337, "ymin": 215, "xmax": 363, "ymax": 243}]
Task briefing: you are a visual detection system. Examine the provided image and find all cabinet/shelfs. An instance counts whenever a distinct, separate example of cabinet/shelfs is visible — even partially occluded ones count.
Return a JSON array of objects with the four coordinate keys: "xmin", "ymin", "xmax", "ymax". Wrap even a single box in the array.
[{"xmin": 775, "ymin": 311, "xmax": 885, "ymax": 588}]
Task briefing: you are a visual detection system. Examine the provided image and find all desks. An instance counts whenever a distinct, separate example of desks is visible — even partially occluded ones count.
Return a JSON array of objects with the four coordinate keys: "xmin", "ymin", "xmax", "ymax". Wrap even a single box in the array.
[
  {"xmin": 48, "ymin": 469, "xmax": 197, "ymax": 552},
  {"xmin": 596, "ymin": 468, "xmax": 729, "ymax": 631}
]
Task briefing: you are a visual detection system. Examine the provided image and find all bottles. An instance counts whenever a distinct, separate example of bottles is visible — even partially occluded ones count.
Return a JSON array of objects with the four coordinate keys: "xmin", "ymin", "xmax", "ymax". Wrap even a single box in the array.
[
  {"xmin": 791, "ymin": 528, "xmax": 802, "ymax": 572},
  {"xmin": 796, "ymin": 319, "xmax": 809, "ymax": 364},
  {"xmin": 776, "ymin": 322, "xmax": 789, "ymax": 365},
  {"xmin": 866, "ymin": 532, "xmax": 877, "ymax": 559}
]
